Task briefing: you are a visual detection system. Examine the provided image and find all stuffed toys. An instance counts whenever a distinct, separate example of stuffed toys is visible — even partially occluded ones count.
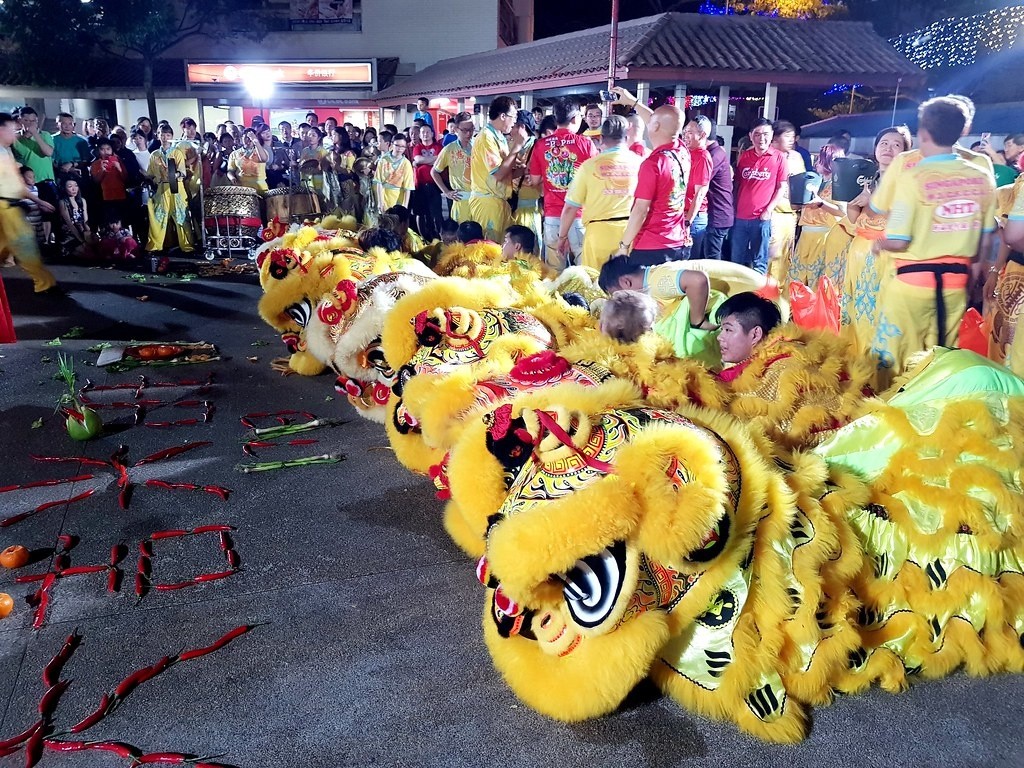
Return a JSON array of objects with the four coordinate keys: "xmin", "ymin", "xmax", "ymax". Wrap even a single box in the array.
[
  {"xmin": 250, "ymin": 216, "xmax": 608, "ymax": 502},
  {"xmin": 475, "ymin": 343, "xmax": 1024, "ymax": 750},
  {"xmin": 386, "ymin": 321, "xmax": 881, "ymax": 567}
]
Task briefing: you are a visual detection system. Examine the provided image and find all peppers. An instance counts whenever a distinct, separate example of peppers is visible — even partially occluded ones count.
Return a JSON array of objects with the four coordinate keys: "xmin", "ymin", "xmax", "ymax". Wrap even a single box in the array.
[{"xmin": 1, "ymin": 374, "xmax": 328, "ymax": 768}]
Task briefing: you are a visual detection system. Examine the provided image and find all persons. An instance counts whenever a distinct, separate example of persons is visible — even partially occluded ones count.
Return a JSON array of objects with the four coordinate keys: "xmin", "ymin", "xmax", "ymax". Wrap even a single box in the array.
[
  {"xmin": 991, "ymin": 169, "xmax": 1024, "ymax": 380},
  {"xmin": 10, "ymin": 86, "xmax": 1024, "ymax": 314},
  {"xmin": 0, "ymin": 113, "xmax": 72, "ymax": 304},
  {"xmin": 600, "ymin": 288, "xmax": 658, "ymax": 345},
  {"xmin": 981, "ymin": 170, "xmax": 1018, "ymax": 368},
  {"xmin": 599, "ymin": 255, "xmax": 792, "ymax": 361},
  {"xmin": 868, "ymin": 97, "xmax": 998, "ymax": 388},
  {"xmin": 714, "ymin": 292, "xmax": 782, "ymax": 363},
  {"xmin": 837, "ymin": 126, "xmax": 912, "ymax": 351}
]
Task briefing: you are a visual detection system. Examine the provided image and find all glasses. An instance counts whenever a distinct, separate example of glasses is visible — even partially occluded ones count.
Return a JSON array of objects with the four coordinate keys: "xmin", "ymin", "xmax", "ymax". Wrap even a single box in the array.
[
  {"xmin": 393, "ymin": 143, "xmax": 406, "ymax": 148},
  {"xmin": 457, "ymin": 126, "xmax": 475, "ymax": 132},
  {"xmin": 505, "ymin": 114, "xmax": 518, "ymax": 119},
  {"xmin": 22, "ymin": 118, "xmax": 37, "ymax": 122},
  {"xmin": 587, "ymin": 115, "xmax": 602, "ymax": 118}
]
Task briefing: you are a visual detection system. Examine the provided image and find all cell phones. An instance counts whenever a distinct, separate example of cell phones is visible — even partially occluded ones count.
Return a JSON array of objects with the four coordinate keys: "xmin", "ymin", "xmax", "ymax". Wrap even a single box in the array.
[
  {"xmin": 599, "ymin": 90, "xmax": 618, "ymax": 103},
  {"xmin": 981, "ymin": 132, "xmax": 991, "ymax": 145},
  {"xmin": 108, "ymin": 155, "xmax": 116, "ymax": 167}
]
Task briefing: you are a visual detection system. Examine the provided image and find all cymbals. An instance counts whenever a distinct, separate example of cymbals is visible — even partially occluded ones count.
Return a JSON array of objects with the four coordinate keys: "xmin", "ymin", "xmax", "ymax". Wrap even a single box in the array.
[
  {"xmin": 297, "ymin": 158, "xmax": 320, "ymax": 174},
  {"xmin": 350, "ymin": 156, "xmax": 376, "ymax": 176},
  {"xmin": 361, "ymin": 144, "xmax": 382, "ymax": 159}
]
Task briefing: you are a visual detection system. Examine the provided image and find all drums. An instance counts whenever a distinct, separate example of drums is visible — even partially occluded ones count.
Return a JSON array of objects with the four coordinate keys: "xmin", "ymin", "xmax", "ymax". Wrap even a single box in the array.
[
  {"xmin": 264, "ymin": 186, "xmax": 323, "ymax": 227},
  {"xmin": 195, "ymin": 185, "xmax": 265, "ymax": 261}
]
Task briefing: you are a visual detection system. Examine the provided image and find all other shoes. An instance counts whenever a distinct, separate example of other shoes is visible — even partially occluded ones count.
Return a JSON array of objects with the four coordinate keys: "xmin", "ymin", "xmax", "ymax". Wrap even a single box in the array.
[
  {"xmin": 185, "ymin": 251, "xmax": 198, "ymax": 257},
  {"xmin": 42, "ymin": 287, "xmax": 69, "ymax": 303},
  {"xmin": 149, "ymin": 251, "xmax": 165, "ymax": 256}
]
